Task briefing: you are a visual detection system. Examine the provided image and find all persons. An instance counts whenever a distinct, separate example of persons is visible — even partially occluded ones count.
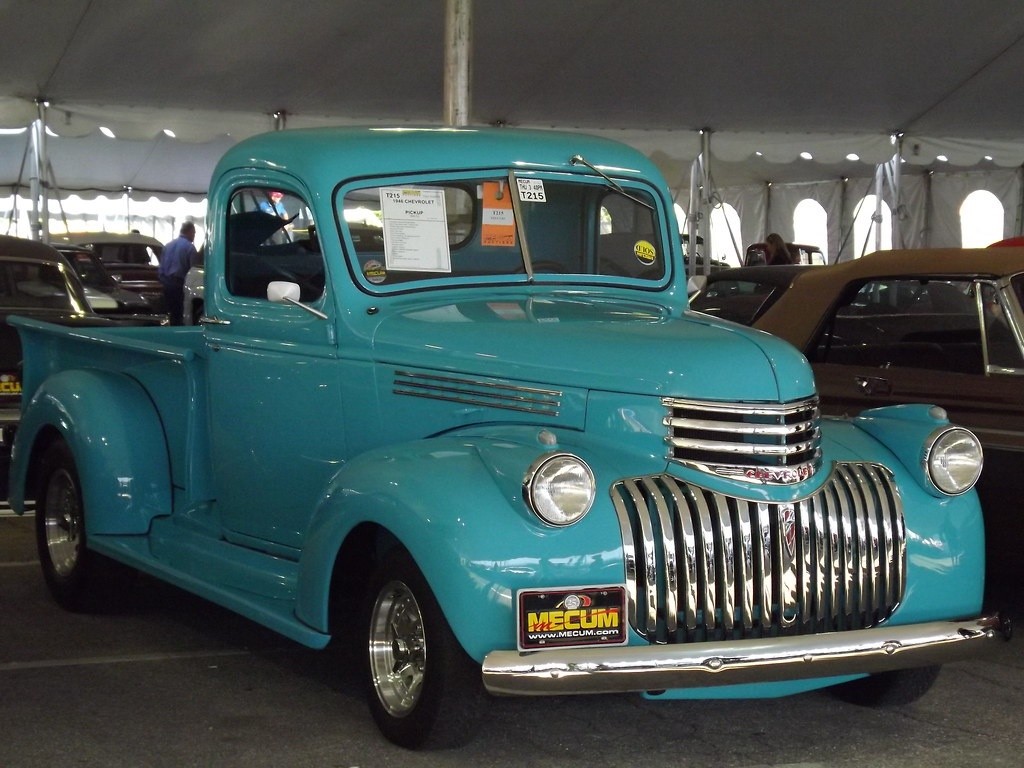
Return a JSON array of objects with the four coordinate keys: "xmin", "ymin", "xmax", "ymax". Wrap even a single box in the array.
[
  {"xmin": 129, "ymin": 230, "xmax": 150, "ymax": 263},
  {"xmin": 157, "ymin": 222, "xmax": 198, "ymax": 325},
  {"xmin": 766, "ymin": 233, "xmax": 794, "ymax": 264},
  {"xmin": 254, "ymin": 190, "xmax": 295, "ymax": 245}
]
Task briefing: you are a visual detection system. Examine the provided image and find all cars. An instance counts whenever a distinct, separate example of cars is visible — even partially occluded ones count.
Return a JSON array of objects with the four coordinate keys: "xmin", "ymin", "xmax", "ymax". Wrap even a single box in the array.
[
  {"xmin": 749, "ymin": 244, "xmax": 1024, "ymax": 487},
  {"xmin": 49, "ymin": 242, "xmax": 152, "ymax": 317},
  {"xmin": 0, "ymin": 235, "xmax": 173, "ymax": 482},
  {"xmin": 683, "ymin": 261, "xmax": 1018, "ymax": 377},
  {"xmin": 39, "ymin": 229, "xmax": 173, "ymax": 318}
]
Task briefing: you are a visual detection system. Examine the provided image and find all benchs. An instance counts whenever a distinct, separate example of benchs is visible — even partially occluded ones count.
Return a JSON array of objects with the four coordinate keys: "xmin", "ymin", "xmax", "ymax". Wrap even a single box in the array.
[{"xmin": 825, "ymin": 339, "xmax": 1021, "ymax": 376}]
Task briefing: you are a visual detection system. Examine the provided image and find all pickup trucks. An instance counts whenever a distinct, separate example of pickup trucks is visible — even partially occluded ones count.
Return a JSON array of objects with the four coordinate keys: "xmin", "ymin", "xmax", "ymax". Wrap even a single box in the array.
[{"xmin": 5, "ymin": 123, "xmax": 1007, "ymax": 749}]
